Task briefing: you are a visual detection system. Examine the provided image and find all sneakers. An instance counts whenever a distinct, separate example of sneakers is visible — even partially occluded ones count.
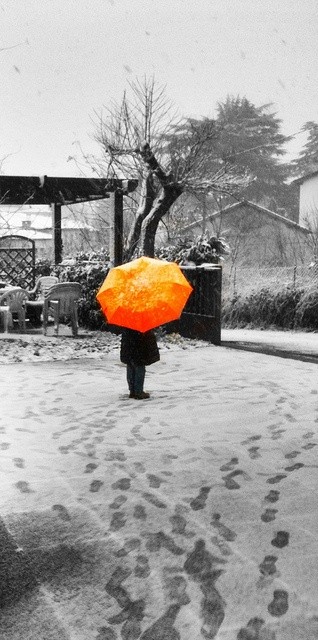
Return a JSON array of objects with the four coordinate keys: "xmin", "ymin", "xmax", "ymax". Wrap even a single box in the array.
[
  {"xmin": 135, "ymin": 393, "xmax": 149, "ymax": 399},
  {"xmin": 130, "ymin": 393, "xmax": 135, "ymax": 398}
]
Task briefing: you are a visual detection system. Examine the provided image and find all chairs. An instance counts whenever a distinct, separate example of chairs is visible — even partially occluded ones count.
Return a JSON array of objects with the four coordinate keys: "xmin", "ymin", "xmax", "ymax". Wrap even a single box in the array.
[
  {"xmin": 0, "ymin": 287, "xmax": 30, "ymax": 334},
  {"xmin": 44, "ymin": 282, "xmax": 81, "ymax": 336},
  {"xmin": 28, "ymin": 276, "xmax": 60, "ymax": 325}
]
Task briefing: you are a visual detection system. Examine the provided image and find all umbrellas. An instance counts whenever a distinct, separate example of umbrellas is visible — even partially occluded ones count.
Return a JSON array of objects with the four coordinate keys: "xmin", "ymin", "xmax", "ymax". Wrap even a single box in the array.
[{"xmin": 96, "ymin": 256, "xmax": 193, "ymax": 333}]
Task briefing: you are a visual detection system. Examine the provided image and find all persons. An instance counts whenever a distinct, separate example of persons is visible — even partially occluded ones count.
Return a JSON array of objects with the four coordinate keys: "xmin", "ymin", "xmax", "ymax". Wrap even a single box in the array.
[
  {"xmin": 120, "ymin": 327, "xmax": 160, "ymax": 399},
  {"xmin": 28, "ymin": 266, "xmax": 58, "ymax": 301}
]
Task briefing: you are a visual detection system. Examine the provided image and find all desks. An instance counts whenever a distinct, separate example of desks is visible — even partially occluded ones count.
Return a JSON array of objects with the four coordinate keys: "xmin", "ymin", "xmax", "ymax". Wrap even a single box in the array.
[{"xmin": 3, "ymin": 301, "xmax": 43, "ymax": 329}]
